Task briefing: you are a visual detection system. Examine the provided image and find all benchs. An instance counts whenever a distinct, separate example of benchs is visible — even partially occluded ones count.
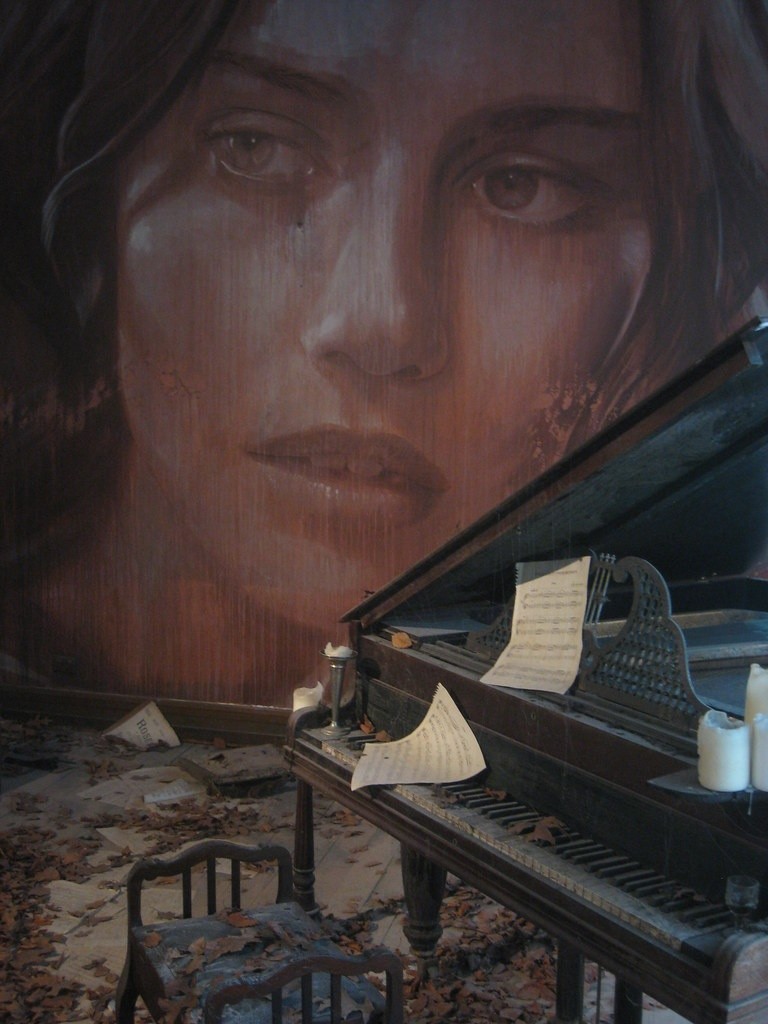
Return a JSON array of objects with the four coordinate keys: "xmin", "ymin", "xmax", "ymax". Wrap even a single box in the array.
[{"xmin": 115, "ymin": 837, "xmax": 403, "ymax": 1024}]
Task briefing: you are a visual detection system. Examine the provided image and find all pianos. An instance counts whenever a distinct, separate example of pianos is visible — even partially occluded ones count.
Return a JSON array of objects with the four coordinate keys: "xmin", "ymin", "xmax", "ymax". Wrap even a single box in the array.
[{"xmin": 278, "ymin": 299, "xmax": 768, "ymax": 1024}]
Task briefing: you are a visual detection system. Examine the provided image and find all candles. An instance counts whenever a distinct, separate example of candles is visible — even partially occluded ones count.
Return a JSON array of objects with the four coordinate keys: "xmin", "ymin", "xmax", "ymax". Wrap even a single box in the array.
[
  {"xmin": 697, "ymin": 709, "xmax": 750, "ymax": 791},
  {"xmin": 325, "ymin": 642, "xmax": 353, "ymax": 657},
  {"xmin": 745, "ymin": 663, "xmax": 768, "ymax": 724},
  {"xmin": 752, "ymin": 713, "xmax": 768, "ymax": 792}
]
